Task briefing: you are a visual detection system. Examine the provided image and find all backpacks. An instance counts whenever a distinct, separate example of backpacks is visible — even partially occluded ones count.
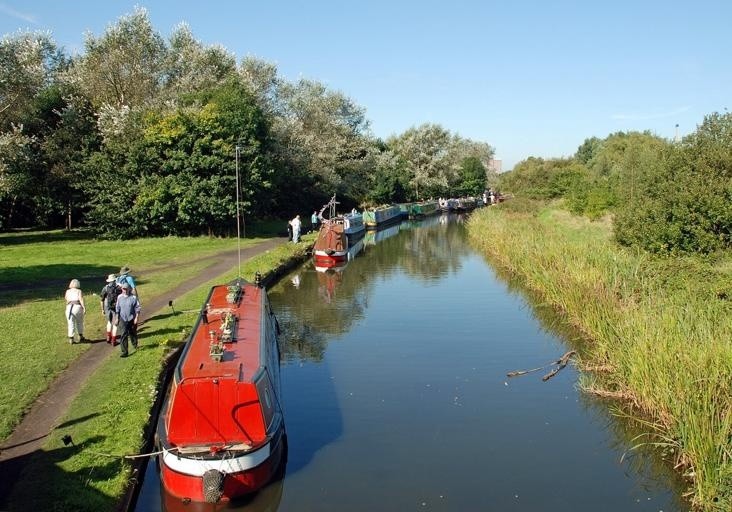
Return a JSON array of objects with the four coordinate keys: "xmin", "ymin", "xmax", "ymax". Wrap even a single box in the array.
[{"xmin": 106, "ymin": 285, "xmax": 118, "ymax": 311}]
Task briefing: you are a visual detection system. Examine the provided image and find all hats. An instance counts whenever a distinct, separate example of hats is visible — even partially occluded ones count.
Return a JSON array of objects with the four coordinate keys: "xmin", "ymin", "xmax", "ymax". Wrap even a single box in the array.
[
  {"xmin": 121, "ymin": 284, "xmax": 132, "ymax": 289},
  {"xmin": 120, "ymin": 266, "xmax": 131, "ymax": 274},
  {"xmin": 105, "ymin": 274, "xmax": 117, "ymax": 283}
]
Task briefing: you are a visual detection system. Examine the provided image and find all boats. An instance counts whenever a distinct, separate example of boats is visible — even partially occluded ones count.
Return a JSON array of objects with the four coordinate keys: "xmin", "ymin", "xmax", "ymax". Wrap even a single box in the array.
[
  {"xmin": 156, "ymin": 144, "xmax": 288, "ymax": 507},
  {"xmin": 312, "ymin": 261, "xmax": 350, "ymax": 304},
  {"xmin": 356, "ymin": 190, "xmax": 509, "ymax": 232},
  {"xmin": 312, "ymin": 192, "xmax": 366, "ymax": 264}
]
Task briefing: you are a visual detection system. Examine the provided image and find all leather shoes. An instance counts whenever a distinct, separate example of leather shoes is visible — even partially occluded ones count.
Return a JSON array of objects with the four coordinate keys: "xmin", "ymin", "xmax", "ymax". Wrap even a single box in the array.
[{"xmin": 120, "ymin": 353, "xmax": 128, "ymax": 357}]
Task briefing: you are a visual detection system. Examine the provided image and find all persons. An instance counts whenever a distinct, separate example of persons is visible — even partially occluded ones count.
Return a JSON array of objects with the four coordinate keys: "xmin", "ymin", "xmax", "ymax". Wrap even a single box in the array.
[
  {"xmin": 64, "ymin": 266, "xmax": 141, "ymax": 358},
  {"xmin": 287, "ymin": 210, "xmax": 318, "ymax": 244}
]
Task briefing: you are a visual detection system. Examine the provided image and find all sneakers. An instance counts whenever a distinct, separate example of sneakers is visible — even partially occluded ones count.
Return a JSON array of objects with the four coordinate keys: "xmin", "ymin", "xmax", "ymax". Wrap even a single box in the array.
[
  {"xmin": 106, "ymin": 337, "xmax": 120, "ymax": 347},
  {"xmin": 69, "ymin": 341, "xmax": 76, "ymax": 345},
  {"xmin": 79, "ymin": 337, "xmax": 89, "ymax": 342}
]
